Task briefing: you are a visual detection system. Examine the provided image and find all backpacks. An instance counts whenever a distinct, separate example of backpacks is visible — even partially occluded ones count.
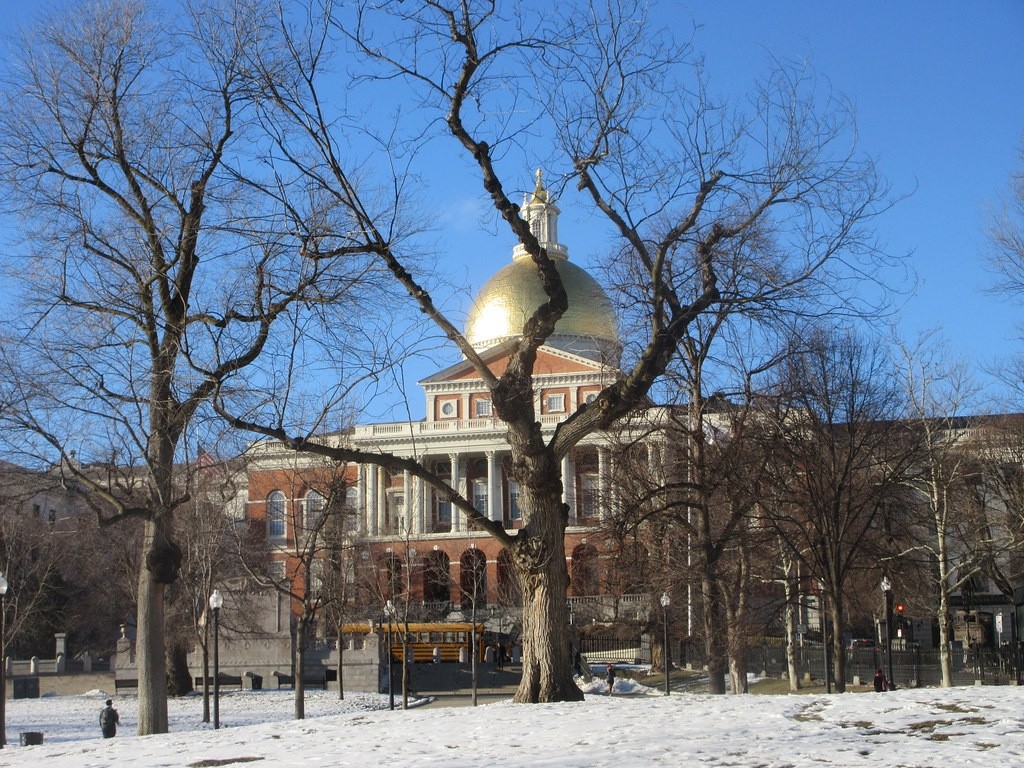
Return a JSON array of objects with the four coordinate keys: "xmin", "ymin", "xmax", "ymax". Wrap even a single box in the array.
[{"xmin": 101, "ymin": 710, "xmax": 114, "ymax": 728}]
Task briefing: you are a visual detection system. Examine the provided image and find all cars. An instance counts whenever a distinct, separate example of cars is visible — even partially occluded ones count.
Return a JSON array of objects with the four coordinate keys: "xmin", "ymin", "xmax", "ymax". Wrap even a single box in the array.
[{"xmin": 851, "ymin": 637, "xmax": 875, "ymax": 652}]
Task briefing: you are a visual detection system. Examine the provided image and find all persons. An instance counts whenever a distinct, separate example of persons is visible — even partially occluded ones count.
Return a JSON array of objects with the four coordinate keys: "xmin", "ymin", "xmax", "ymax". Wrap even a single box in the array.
[
  {"xmin": 495, "ymin": 642, "xmax": 507, "ymax": 671},
  {"xmin": 99, "ymin": 700, "xmax": 120, "ymax": 739},
  {"xmin": 604, "ymin": 664, "xmax": 615, "ymax": 696},
  {"xmin": 969, "ymin": 638, "xmax": 1024, "ymax": 673},
  {"xmin": 873, "ymin": 669, "xmax": 889, "ymax": 693}
]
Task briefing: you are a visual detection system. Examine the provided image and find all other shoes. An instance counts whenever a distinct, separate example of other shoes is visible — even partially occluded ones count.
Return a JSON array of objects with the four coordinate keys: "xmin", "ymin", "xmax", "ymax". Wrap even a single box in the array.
[{"xmin": 610, "ymin": 693, "xmax": 613, "ymax": 697}]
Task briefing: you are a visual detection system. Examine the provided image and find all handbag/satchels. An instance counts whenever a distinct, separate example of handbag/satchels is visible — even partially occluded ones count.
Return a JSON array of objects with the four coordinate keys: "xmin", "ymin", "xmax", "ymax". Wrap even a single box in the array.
[{"xmin": 882, "ymin": 679, "xmax": 886, "ymax": 690}]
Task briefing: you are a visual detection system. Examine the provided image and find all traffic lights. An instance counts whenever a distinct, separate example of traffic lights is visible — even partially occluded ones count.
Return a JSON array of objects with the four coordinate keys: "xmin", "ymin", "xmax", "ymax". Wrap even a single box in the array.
[{"xmin": 898, "ymin": 605, "xmax": 904, "ymax": 611}]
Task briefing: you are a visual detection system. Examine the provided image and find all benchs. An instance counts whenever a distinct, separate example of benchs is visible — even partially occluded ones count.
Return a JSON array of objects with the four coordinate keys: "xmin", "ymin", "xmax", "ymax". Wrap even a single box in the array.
[
  {"xmin": 195, "ymin": 677, "xmax": 243, "ymax": 690},
  {"xmin": 278, "ymin": 674, "xmax": 326, "ymax": 689},
  {"xmin": 115, "ymin": 678, "xmax": 138, "ymax": 693}
]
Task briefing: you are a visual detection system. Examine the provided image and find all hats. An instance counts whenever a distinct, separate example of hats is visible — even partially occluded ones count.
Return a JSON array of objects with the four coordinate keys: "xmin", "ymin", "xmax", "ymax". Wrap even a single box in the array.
[{"xmin": 607, "ymin": 663, "xmax": 611, "ymax": 667}]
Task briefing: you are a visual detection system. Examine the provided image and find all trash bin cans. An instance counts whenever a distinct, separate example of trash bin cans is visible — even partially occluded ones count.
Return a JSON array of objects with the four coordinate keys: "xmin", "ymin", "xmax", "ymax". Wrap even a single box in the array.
[{"xmin": 13, "ymin": 678, "xmax": 40, "ymax": 698}]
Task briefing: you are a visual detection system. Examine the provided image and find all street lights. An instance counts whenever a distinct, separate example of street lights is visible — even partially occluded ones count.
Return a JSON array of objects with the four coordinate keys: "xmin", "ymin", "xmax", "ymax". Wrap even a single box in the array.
[
  {"xmin": 660, "ymin": 591, "xmax": 672, "ymax": 696},
  {"xmin": 209, "ymin": 589, "xmax": 225, "ymax": 731},
  {"xmin": 0, "ymin": 572, "xmax": 10, "ymax": 749},
  {"xmin": 880, "ymin": 575, "xmax": 896, "ymax": 691},
  {"xmin": 383, "ymin": 599, "xmax": 397, "ymax": 711},
  {"xmin": 818, "ymin": 577, "xmax": 831, "ymax": 694}
]
